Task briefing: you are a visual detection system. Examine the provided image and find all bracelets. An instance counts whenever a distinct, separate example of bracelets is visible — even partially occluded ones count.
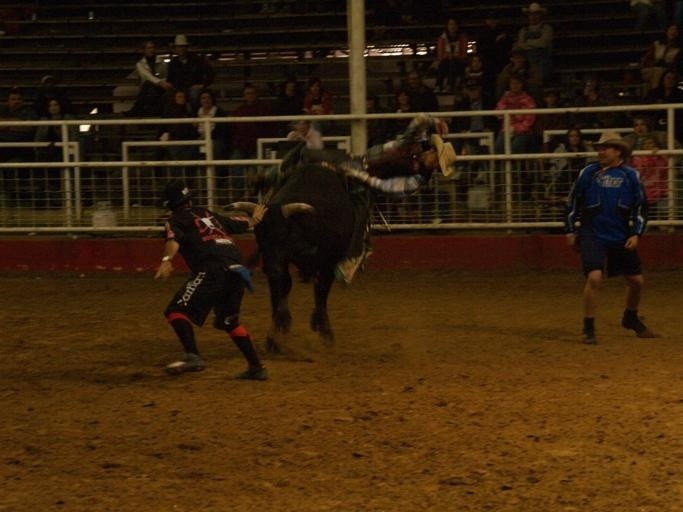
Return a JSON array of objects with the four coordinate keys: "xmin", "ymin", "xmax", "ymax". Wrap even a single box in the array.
[
  {"xmin": 251, "ymin": 215, "xmax": 261, "ymax": 223},
  {"xmin": 161, "ymin": 255, "xmax": 174, "ymax": 262}
]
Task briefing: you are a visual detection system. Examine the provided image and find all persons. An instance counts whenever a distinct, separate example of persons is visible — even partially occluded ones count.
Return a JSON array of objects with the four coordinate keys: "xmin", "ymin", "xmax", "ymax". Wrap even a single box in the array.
[
  {"xmin": 1, "ymin": 3, "xmax": 682, "ymax": 220},
  {"xmin": 151, "ymin": 179, "xmax": 275, "ymax": 378},
  {"xmin": 564, "ymin": 130, "xmax": 652, "ymax": 342}
]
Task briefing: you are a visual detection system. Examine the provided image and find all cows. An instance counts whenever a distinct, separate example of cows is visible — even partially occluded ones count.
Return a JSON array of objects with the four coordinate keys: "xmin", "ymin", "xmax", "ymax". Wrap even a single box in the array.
[{"xmin": 221, "ymin": 146, "xmax": 375, "ymax": 353}]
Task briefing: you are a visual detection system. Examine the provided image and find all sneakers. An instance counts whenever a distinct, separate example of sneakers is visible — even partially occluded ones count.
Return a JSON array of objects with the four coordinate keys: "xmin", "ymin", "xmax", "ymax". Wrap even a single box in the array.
[
  {"xmin": 620, "ymin": 312, "xmax": 657, "ymax": 339},
  {"xmin": 162, "ymin": 350, "xmax": 208, "ymax": 376},
  {"xmin": 581, "ymin": 329, "xmax": 600, "ymax": 346},
  {"xmin": 233, "ymin": 362, "xmax": 270, "ymax": 382}
]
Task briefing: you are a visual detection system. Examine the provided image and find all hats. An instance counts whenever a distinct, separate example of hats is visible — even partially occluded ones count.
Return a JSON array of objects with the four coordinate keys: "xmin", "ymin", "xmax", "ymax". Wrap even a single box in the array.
[
  {"xmin": 154, "ymin": 179, "xmax": 193, "ymax": 211},
  {"xmin": 520, "ymin": 0, "xmax": 549, "ymax": 17},
  {"xmin": 168, "ymin": 32, "xmax": 193, "ymax": 49},
  {"xmin": 427, "ymin": 132, "xmax": 461, "ymax": 179},
  {"xmin": 592, "ymin": 131, "xmax": 632, "ymax": 152}
]
